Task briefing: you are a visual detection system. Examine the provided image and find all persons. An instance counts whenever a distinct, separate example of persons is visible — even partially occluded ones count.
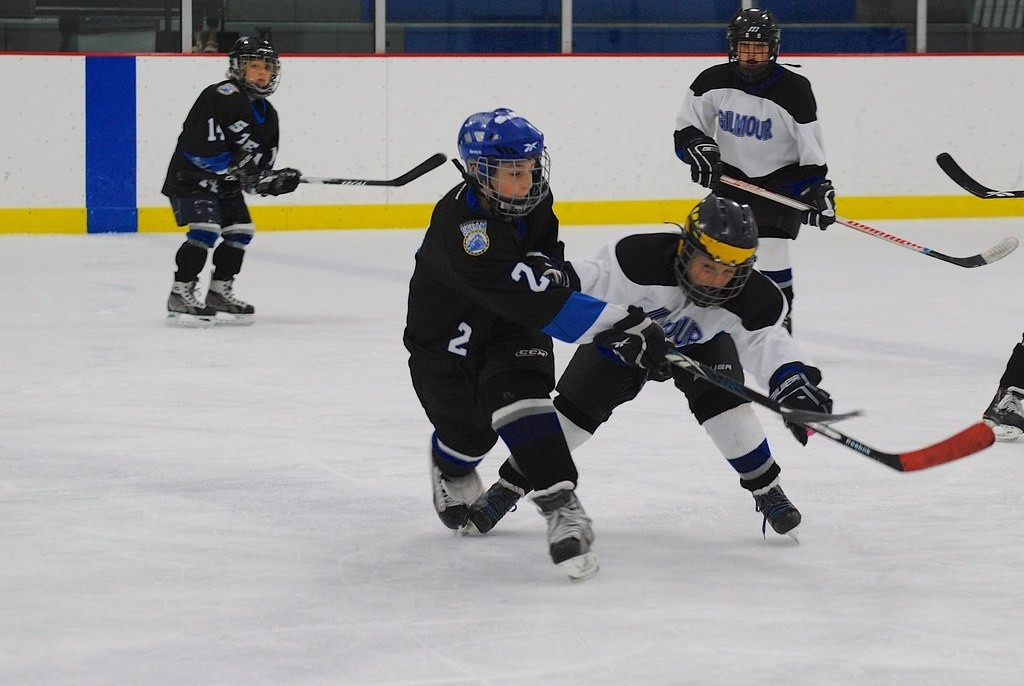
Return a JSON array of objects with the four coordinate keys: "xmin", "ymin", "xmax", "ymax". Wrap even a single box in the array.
[
  {"xmin": 192, "ymin": 0, "xmax": 222, "ymax": 52},
  {"xmin": 462, "ymin": 193, "xmax": 831, "ymax": 546},
  {"xmin": 403, "ymin": 109, "xmax": 676, "ymax": 583},
  {"xmin": 982, "ymin": 332, "xmax": 1024, "ymax": 443},
  {"xmin": 673, "ymin": 7, "xmax": 835, "ymax": 335},
  {"xmin": 160, "ymin": 36, "xmax": 301, "ymax": 328}
]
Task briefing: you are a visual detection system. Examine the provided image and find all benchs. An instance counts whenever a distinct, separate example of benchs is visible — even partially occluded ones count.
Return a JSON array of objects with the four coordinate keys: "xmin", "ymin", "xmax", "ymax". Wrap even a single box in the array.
[{"xmin": 0, "ymin": 0, "xmax": 976, "ymax": 51}]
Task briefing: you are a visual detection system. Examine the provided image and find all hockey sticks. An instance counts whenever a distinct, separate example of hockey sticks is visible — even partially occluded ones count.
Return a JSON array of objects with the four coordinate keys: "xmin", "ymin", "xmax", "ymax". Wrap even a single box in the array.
[
  {"xmin": 259, "ymin": 152, "xmax": 449, "ymax": 189},
  {"xmin": 665, "ymin": 349, "xmax": 998, "ymax": 475},
  {"xmin": 935, "ymin": 150, "xmax": 1024, "ymax": 201},
  {"xmin": 718, "ymin": 173, "xmax": 1020, "ymax": 269}
]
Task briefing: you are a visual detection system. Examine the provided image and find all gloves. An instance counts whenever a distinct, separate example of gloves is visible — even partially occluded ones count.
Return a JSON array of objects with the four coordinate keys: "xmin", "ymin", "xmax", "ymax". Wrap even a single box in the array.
[
  {"xmin": 800, "ymin": 180, "xmax": 836, "ymax": 230},
  {"xmin": 595, "ymin": 303, "xmax": 675, "ymax": 383},
  {"xmin": 768, "ymin": 363, "xmax": 833, "ymax": 444},
  {"xmin": 257, "ymin": 167, "xmax": 301, "ymax": 196},
  {"xmin": 537, "ymin": 259, "xmax": 580, "ymax": 291},
  {"xmin": 674, "ymin": 127, "xmax": 723, "ymax": 190}
]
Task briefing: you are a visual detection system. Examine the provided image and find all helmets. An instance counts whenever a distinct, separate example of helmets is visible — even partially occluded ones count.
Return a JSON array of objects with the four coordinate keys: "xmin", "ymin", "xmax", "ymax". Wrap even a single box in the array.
[
  {"xmin": 452, "ymin": 108, "xmax": 551, "ymax": 217},
  {"xmin": 226, "ymin": 37, "xmax": 281, "ymax": 100},
  {"xmin": 663, "ymin": 194, "xmax": 757, "ymax": 309},
  {"xmin": 728, "ymin": 9, "xmax": 781, "ymax": 82}
]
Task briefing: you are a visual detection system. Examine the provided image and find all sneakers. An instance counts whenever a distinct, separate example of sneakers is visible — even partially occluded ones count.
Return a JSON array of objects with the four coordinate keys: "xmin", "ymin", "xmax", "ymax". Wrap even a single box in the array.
[
  {"xmin": 431, "ymin": 463, "xmax": 467, "ymax": 530},
  {"xmin": 753, "ymin": 487, "xmax": 802, "ymax": 535},
  {"xmin": 534, "ymin": 496, "xmax": 593, "ymax": 564},
  {"xmin": 166, "ymin": 283, "xmax": 215, "ymax": 316},
  {"xmin": 204, "ymin": 279, "xmax": 257, "ymax": 315},
  {"xmin": 467, "ymin": 483, "xmax": 522, "ymax": 533},
  {"xmin": 985, "ymin": 388, "xmax": 1024, "ymax": 443}
]
